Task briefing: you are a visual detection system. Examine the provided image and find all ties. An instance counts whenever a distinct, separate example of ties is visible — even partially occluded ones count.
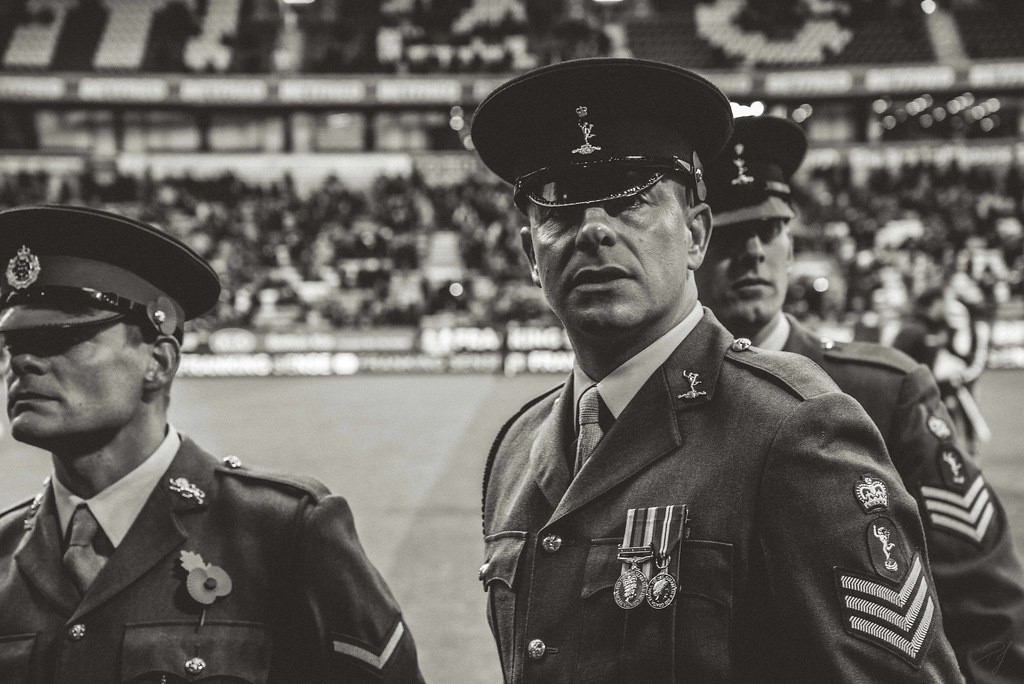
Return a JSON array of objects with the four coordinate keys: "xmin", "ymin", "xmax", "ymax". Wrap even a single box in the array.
[
  {"xmin": 570, "ymin": 387, "xmax": 609, "ymax": 479},
  {"xmin": 62, "ymin": 505, "xmax": 100, "ymax": 595}
]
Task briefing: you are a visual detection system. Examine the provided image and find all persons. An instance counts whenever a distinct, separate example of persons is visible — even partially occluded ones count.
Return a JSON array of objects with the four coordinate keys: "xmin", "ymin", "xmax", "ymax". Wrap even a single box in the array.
[
  {"xmin": 0, "ymin": 204, "xmax": 428, "ymax": 684},
  {"xmin": 471, "ymin": 57, "xmax": 965, "ymax": 684},
  {"xmin": 0, "ymin": 0, "xmax": 1024, "ymax": 447},
  {"xmin": 698, "ymin": 118, "xmax": 1024, "ymax": 684}
]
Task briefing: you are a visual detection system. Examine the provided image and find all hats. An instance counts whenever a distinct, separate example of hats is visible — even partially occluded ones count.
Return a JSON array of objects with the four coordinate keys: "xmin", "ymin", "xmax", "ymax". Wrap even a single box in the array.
[
  {"xmin": 0, "ymin": 204, "xmax": 222, "ymax": 347},
  {"xmin": 706, "ymin": 115, "xmax": 808, "ymax": 230},
  {"xmin": 470, "ymin": 58, "xmax": 734, "ymax": 217}
]
